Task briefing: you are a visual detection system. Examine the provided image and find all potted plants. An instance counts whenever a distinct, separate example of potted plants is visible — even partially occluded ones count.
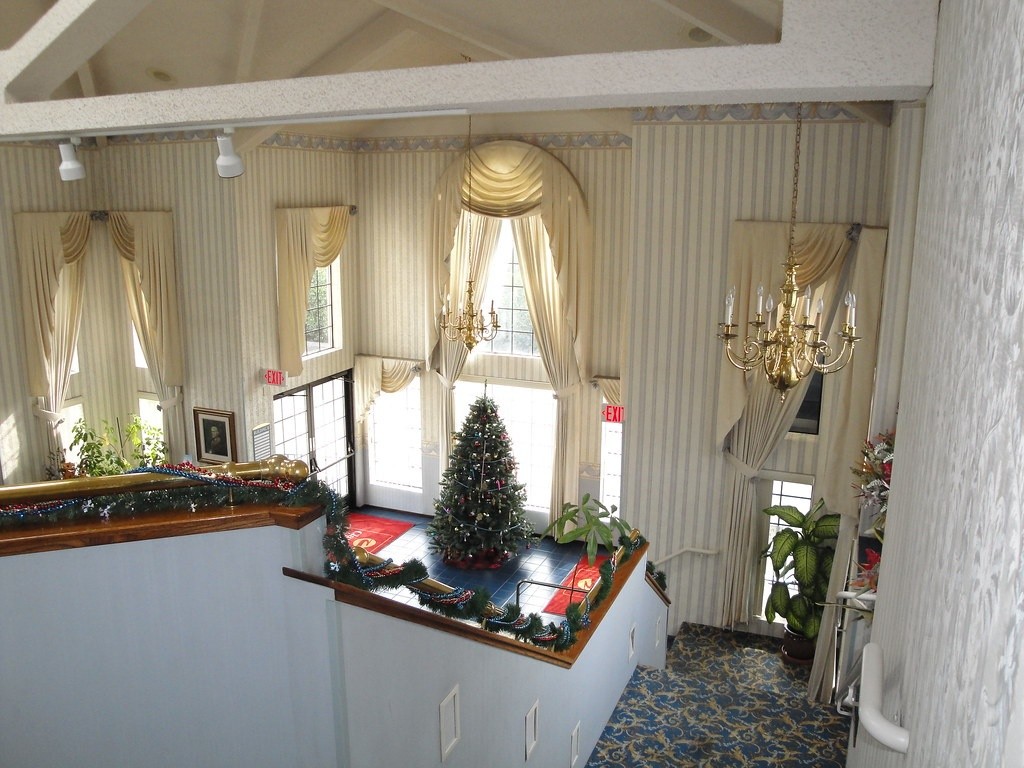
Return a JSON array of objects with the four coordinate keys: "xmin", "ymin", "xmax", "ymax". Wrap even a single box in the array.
[{"xmin": 760, "ymin": 497, "xmax": 842, "ymax": 663}]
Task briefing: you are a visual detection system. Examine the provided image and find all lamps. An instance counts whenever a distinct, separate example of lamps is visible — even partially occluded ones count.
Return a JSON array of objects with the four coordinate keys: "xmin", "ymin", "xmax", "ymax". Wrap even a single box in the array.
[
  {"xmin": 58, "ymin": 144, "xmax": 86, "ymax": 181},
  {"xmin": 717, "ymin": 261, "xmax": 861, "ymax": 403},
  {"xmin": 215, "ymin": 126, "xmax": 244, "ymax": 179},
  {"xmin": 434, "ymin": 280, "xmax": 502, "ymax": 353}
]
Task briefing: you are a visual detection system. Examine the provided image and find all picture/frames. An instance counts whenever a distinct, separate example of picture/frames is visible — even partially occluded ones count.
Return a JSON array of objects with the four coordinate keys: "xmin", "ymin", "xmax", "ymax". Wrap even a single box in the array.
[{"xmin": 193, "ymin": 407, "xmax": 238, "ymax": 465}]
